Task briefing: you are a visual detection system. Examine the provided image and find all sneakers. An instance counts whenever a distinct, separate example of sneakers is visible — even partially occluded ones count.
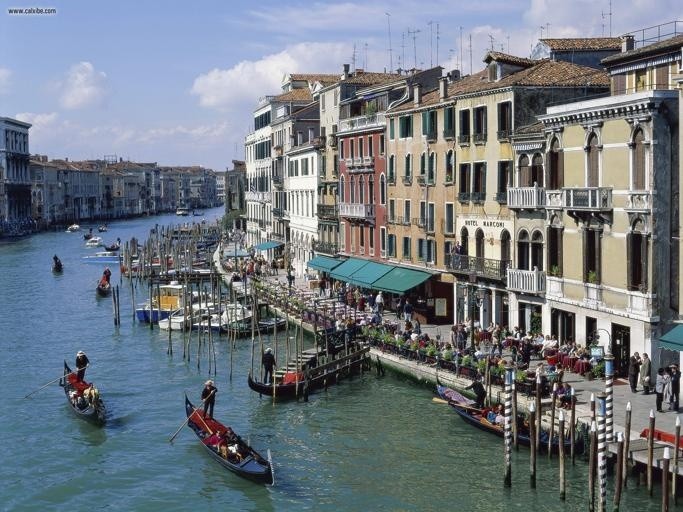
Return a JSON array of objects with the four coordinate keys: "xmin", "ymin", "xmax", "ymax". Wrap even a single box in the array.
[{"xmin": 631, "ymin": 386, "xmax": 680, "ymax": 415}]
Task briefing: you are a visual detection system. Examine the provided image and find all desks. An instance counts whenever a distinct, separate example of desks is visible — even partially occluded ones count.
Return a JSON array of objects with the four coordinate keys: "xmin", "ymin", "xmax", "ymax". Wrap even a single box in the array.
[{"xmin": 475, "ymin": 321, "xmax": 592, "ymax": 375}]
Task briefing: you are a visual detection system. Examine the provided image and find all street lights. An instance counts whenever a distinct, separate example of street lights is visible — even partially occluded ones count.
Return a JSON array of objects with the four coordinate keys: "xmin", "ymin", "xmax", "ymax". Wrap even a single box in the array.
[
  {"xmin": 459, "ymin": 268, "xmax": 487, "ymax": 358},
  {"xmin": 280, "ymin": 244, "xmax": 295, "ymax": 296}
]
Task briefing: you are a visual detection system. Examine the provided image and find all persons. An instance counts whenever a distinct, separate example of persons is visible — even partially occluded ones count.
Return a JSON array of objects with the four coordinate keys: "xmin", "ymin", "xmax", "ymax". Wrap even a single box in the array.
[
  {"xmin": 655, "ymin": 368, "xmax": 668, "ymax": 413},
  {"xmin": 227, "ymin": 244, "xmax": 279, "ymax": 277},
  {"xmin": 449, "ymin": 317, "xmax": 590, "ymax": 412},
  {"xmin": 639, "ymin": 352, "xmax": 651, "ymax": 395},
  {"xmin": 103, "ymin": 266, "xmax": 111, "ymax": 282},
  {"xmin": 199, "ymin": 380, "xmax": 218, "ymax": 420},
  {"xmin": 627, "ymin": 352, "xmax": 642, "ymax": 394},
  {"xmin": 290, "ymin": 268, "xmax": 295, "ymax": 286},
  {"xmin": 74, "ymin": 350, "xmax": 89, "ymax": 381},
  {"xmin": 317, "ymin": 277, "xmax": 448, "ymax": 354},
  {"xmin": 262, "ymin": 347, "xmax": 277, "ymax": 384},
  {"xmin": 667, "ymin": 365, "xmax": 681, "ymax": 411},
  {"xmin": 224, "ymin": 427, "xmax": 238, "ymax": 444},
  {"xmin": 209, "ymin": 429, "xmax": 226, "ymax": 450}
]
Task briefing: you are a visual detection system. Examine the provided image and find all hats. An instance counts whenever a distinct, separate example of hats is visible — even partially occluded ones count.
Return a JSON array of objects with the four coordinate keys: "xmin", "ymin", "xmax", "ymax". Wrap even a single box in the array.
[
  {"xmin": 205, "ymin": 380, "xmax": 214, "ymax": 386},
  {"xmin": 264, "ymin": 347, "xmax": 272, "ymax": 352},
  {"xmin": 76, "ymin": 351, "xmax": 86, "ymax": 356}
]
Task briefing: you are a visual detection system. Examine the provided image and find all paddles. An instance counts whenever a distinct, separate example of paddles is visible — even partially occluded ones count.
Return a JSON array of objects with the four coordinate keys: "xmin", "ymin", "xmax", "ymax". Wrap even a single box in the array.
[{"xmin": 433, "ymin": 397, "xmax": 483, "ymax": 412}]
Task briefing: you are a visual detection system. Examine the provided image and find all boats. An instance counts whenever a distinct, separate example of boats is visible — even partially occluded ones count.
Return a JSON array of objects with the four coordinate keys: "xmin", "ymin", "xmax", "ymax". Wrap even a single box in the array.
[
  {"xmin": 61, "ymin": 358, "xmax": 106, "ymax": 425},
  {"xmin": 246, "ymin": 341, "xmax": 363, "ymax": 399},
  {"xmin": 181, "ymin": 394, "xmax": 273, "ymax": 485},
  {"xmin": 175, "ymin": 208, "xmax": 190, "ymax": 216},
  {"xmin": 50, "ymin": 262, "xmax": 64, "ymax": 272},
  {"xmin": 133, "ymin": 279, "xmax": 288, "ymax": 339},
  {"xmin": 83, "ymin": 224, "xmax": 223, "ymax": 284},
  {"xmin": 65, "ymin": 223, "xmax": 80, "ymax": 233},
  {"xmin": 192, "ymin": 212, "xmax": 204, "ymax": 217},
  {"xmin": 95, "ymin": 280, "xmax": 111, "ymax": 296},
  {"xmin": 435, "ymin": 375, "xmax": 583, "ymax": 454}
]
